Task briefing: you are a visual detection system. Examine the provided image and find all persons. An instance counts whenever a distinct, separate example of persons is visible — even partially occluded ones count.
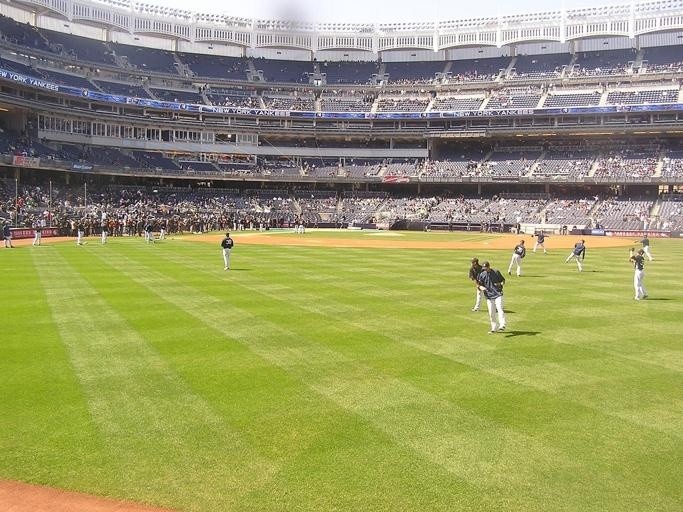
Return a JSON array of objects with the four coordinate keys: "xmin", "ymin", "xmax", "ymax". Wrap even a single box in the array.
[
  {"xmin": 477, "ymin": 261, "xmax": 506, "ymax": 335},
  {"xmin": 531, "ymin": 230, "xmax": 547, "ymax": 255},
  {"xmin": 0, "ymin": 45, "xmax": 682, "ymax": 112},
  {"xmin": 629, "ymin": 247, "xmax": 648, "ymax": 301},
  {"xmin": 564, "ymin": 240, "xmax": 585, "ymax": 272},
  {"xmin": 1, "ymin": 118, "xmax": 682, "ymax": 247},
  {"xmin": 468, "ymin": 257, "xmax": 483, "ymax": 312},
  {"xmin": 221, "ymin": 233, "xmax": 233, "ymax": 272},
  {"xmin": 634, "ymin": 235, "xmax": 653, "ymax": 261},
  {"xmin": 507, "ymin": 240, "xmax": 526, "ymax": 277}
]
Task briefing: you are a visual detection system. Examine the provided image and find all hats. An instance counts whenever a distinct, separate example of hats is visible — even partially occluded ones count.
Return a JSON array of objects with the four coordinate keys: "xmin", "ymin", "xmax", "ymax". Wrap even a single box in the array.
[
  {"xmin": 471, "ymin": 257, "xmax": 479, "ymax": 263},
  {"xmin": 481, "ymin": 261, "xmax": 490, "ymax": 270}
]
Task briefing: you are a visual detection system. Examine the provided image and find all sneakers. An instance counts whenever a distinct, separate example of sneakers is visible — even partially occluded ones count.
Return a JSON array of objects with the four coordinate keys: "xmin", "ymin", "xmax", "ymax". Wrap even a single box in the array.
[{"xmin": 472, "ymin": 308, "xmax": 506, "ymax": 335}]
